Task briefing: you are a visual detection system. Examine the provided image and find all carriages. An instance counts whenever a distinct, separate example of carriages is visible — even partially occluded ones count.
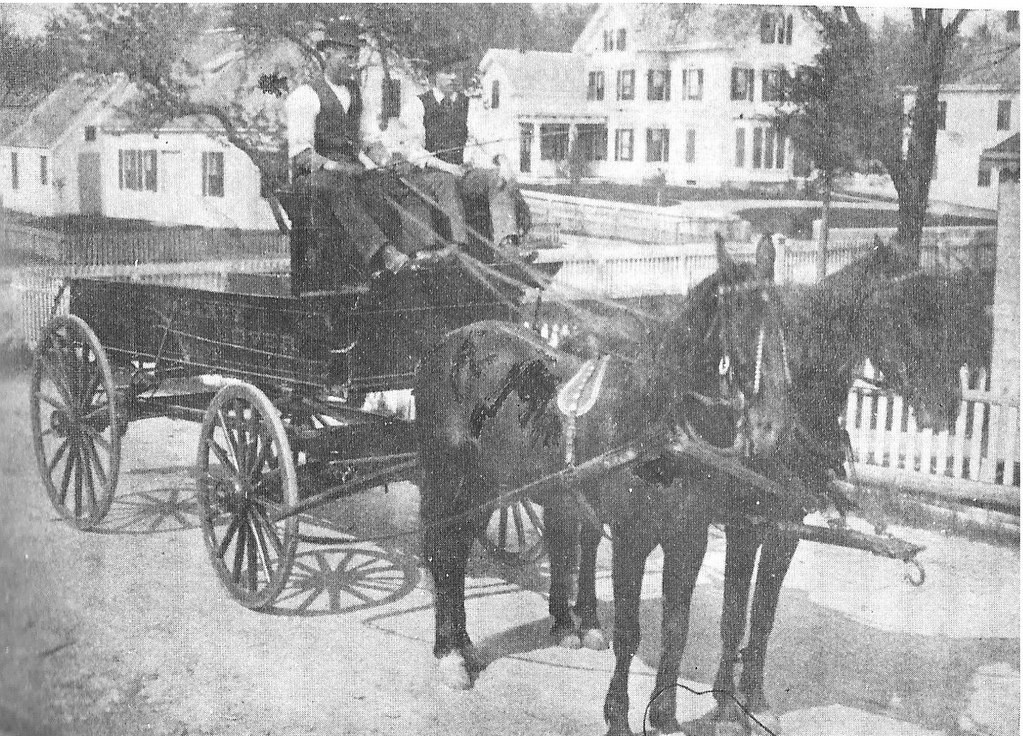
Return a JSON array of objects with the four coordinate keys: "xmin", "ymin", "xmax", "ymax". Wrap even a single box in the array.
[{"xmin": 30, "ymin": 162, "xmax": 970, "ymax": 736}]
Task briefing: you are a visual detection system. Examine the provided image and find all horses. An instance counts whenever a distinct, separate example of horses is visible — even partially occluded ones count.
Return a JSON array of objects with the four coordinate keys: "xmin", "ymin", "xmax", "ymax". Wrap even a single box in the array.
[{"xmin": 413, "ymin": 232, "xmax": 977, "ymax": 731}]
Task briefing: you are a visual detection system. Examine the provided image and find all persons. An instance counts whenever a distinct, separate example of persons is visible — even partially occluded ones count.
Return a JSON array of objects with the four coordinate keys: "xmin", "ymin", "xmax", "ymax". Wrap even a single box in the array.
[
  {"xmin": 397, "ymin": 41, "xmax": 541, "ymax": 272},
  {"xmin": 284, "ymin": 19, "xmax": 413, "ymax": 287},
  {"xmin": 563, "ymin": 133, "xmax": 586, "ymax": 186}
]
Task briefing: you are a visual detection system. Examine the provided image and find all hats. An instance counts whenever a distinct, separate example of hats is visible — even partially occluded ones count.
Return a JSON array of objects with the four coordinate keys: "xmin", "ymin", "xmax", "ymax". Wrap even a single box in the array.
[
  {"xmin": 316, "ymin": 20, "xmax": 366, "ymax": 51},
  {"xmin": 423, "ymin": 43, "xmax": 470, "ymax": 71}
]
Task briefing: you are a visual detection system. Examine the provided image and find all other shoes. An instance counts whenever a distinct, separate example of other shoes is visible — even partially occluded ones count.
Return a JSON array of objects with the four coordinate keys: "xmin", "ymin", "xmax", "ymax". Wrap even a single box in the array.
[
  {"xmin": 414, "ymin": 251, "xmax": 443, "ymax": 268},
  {"xmin": 494, "ymin": 245, "xmax": 539, "ymax": 269},
  {"xmin": 381, "ymin": 245, "xmax": 409, "ymax": 276},
  {"xmin": 443, "ymin": 242, "xmax": 469, "ymax": 264}
]
